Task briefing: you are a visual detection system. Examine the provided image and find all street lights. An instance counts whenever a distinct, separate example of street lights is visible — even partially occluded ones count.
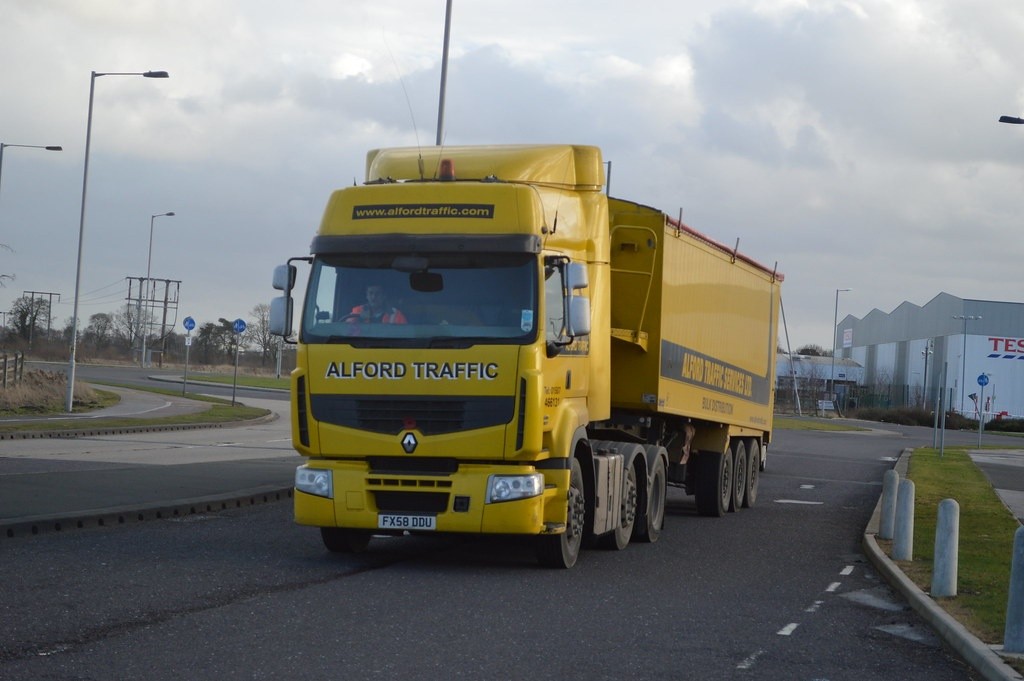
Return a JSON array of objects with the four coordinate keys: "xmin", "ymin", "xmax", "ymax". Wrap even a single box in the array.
[
  {"xmin": 0, "ymin": 143, "xmax": 62, "ymax": 204},
  {"xmin": 828, "ymin": 288, "xmax": 851, "ymax": 402},
  {"xmin": 139, "ymin": 211, "xmax": 177, "ymax": 369},
  {"xmin": 951, "ymin": 314, "xmax": 984, "ymax": 416},
  {"xmin": 63, "ymin": 67, "xmax": 171, "ymax": 411}
]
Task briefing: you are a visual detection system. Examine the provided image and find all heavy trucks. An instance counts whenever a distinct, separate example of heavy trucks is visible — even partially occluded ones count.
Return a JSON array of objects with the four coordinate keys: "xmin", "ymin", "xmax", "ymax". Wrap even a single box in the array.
[{"xmin": 264, "ymin": 144, "xmax": 787, "ymax": 568}]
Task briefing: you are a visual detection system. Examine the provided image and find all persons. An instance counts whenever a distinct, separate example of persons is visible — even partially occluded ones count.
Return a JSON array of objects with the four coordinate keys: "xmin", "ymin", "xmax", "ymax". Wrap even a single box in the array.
[{"xmin": 345, "ymin": 279, "xmax": 407, "ymax": 324}]
